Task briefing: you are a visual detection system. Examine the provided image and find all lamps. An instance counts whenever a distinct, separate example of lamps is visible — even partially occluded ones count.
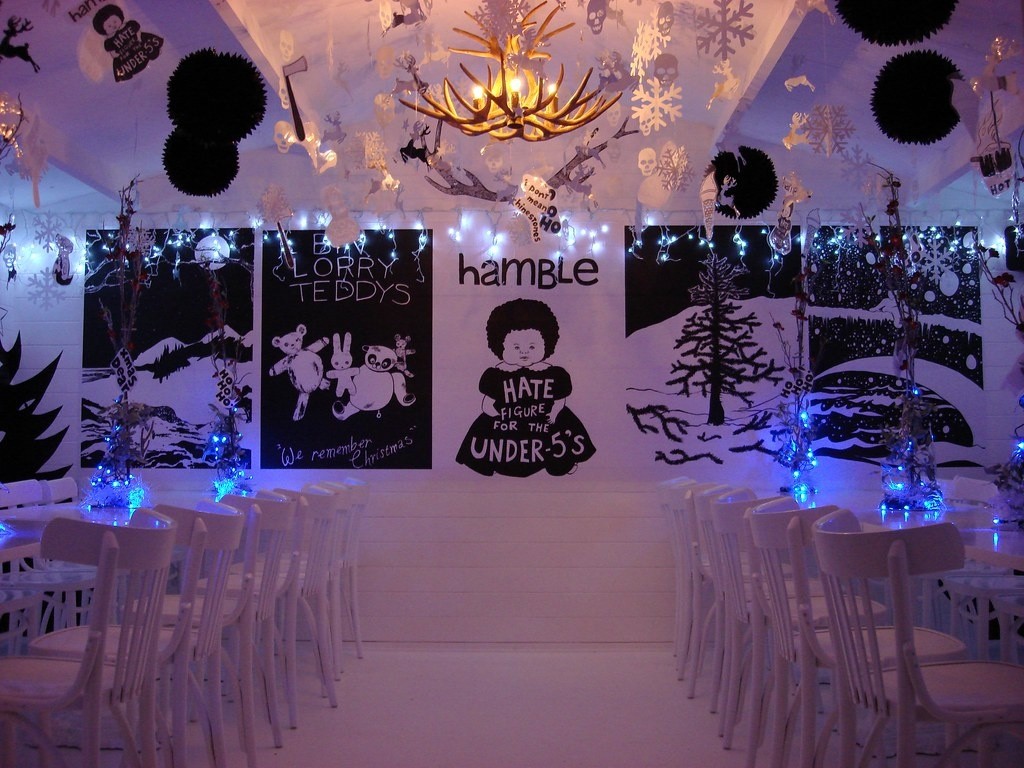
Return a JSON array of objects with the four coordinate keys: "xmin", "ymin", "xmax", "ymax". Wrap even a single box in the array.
[{"xmin": 397, "ymin": 1, "xmax": 622, "ymax": 143}]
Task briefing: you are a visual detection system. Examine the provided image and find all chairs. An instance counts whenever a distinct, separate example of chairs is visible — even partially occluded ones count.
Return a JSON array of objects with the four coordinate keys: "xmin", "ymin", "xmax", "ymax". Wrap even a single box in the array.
[
  {"xmin": 0, "ymin": 475, "xmax": 367, "ymax": 768},
  {"xmin": 660, "ymin": 476, "xmax": 1024, "ymax": 768}
]
